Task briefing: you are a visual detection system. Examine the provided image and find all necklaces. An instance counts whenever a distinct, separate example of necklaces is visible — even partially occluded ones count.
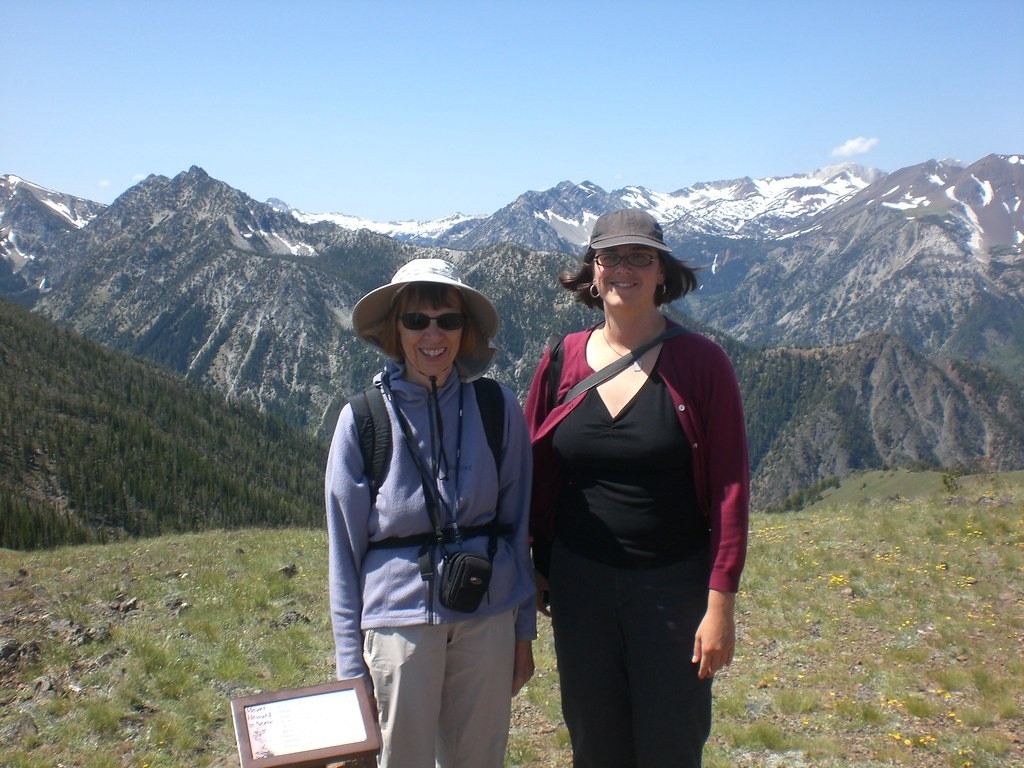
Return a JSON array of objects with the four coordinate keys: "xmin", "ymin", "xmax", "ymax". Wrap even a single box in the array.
[{"xmin": 599, "ymin": 331, "xmax": 661, "ymax": 370}]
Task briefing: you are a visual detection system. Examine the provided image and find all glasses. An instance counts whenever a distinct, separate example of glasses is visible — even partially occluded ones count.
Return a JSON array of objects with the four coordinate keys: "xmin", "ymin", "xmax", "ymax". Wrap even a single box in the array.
[
  {"xmin": 397, "ymin": 312, "xmax": 467, "ymax": 331},
  {"xmin": 591, "ymin": 253, "xmax": 660, "ymax": 267}
]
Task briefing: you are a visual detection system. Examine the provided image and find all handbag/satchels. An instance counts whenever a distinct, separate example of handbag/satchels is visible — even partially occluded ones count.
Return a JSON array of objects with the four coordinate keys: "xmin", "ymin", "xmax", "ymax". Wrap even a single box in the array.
[{"xmin": 440, "ymin": 553, "xmax": 490, "ymax": 613}]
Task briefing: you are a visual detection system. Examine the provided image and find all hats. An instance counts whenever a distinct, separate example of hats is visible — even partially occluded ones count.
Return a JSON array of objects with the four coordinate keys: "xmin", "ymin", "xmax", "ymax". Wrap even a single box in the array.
[
  {"xmin": 352, "ymin": 259, "xmax": 498, "ymax": 362},
  {"xmin": 588, "ymin": 210, "xmax": 673, "ymax": 251}
]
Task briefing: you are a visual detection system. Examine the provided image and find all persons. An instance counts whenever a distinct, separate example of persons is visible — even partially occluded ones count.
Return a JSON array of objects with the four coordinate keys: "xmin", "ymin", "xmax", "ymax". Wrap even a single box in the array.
[
  {"xmin": 324, "ymin": 258, "xmax": 539, "ymax": 768},
  {"xmin": 526, "ymin": 207, "xmax": 752, "ymax": 767}
]
